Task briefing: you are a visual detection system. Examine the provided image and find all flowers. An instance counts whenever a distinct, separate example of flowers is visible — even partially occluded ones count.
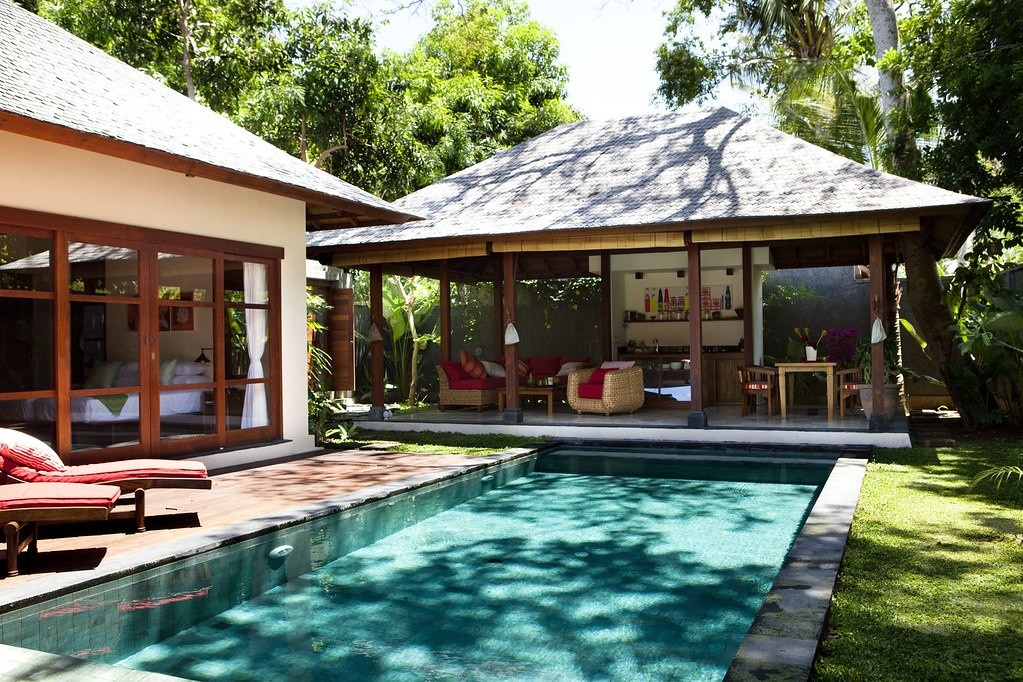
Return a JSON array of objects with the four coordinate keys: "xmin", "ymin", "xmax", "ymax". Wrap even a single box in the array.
[
  {"xmin": 795, "ymin": 327, "xmax": 828, "ymax": 350},
  {"xmin": 774, "ymin": 362, "xmax": 837, "ymax": 422}
]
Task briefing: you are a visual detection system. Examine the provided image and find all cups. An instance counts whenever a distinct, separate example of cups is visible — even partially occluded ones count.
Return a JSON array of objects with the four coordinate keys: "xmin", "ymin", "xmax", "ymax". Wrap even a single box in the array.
[{"xmin": 547, "ymin": 377, "xmax": 553, "ymax": 385}]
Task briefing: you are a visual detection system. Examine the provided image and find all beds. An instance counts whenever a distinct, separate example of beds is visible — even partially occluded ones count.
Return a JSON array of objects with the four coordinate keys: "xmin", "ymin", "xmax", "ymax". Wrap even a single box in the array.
[{"xmin": 0, "ymin": 388, "xmax": 212, "ymax": 447}]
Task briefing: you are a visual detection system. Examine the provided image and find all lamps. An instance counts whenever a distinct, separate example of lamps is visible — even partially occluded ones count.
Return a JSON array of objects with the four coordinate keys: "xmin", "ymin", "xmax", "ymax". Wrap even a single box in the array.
[{"xmin": 194, "ymin": 347, "xmax": 240, "ymax": 390}]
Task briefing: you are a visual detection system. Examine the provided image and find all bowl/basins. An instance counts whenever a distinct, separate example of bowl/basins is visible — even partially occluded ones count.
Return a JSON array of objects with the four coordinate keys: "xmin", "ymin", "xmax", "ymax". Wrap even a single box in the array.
[{"xmin": 658, "ymin": 361, "xmax": 681, "ymax": 370}]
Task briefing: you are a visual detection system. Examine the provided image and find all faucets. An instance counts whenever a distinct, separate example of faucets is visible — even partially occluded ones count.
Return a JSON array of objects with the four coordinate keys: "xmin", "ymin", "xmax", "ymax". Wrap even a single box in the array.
[{"xmin": 653, "ymin": 338, "xmax": 659, "ymax": 353}]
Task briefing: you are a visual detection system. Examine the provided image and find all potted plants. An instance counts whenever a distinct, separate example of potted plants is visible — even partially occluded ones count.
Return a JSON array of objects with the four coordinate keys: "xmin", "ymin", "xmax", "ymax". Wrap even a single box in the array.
[{"xmin": 851, "ymin": 332, "xmax": 947, "ymax": 425}]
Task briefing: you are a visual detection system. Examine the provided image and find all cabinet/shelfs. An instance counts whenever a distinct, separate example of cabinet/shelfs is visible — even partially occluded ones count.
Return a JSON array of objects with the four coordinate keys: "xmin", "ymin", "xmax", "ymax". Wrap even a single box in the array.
[{"xmin": 618, "ymin": 352, "xmax": 745, "ymax": 410}]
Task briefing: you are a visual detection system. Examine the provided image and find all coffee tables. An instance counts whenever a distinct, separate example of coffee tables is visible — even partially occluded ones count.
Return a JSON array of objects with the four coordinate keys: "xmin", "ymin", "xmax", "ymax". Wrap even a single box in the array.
[{"xmin": 496, "ymin": 384, "xmax": 567, "ymax": 416}]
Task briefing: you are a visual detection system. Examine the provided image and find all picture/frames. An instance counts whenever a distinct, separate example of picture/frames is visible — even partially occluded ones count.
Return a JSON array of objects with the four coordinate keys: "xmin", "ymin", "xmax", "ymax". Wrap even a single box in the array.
[{"xmin": 125, "ymin": 291, "xmax": 197, "ymax": 334}]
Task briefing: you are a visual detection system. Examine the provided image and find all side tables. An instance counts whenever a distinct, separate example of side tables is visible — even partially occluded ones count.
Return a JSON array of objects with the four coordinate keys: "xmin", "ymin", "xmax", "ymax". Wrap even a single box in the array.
[{"xmin": 200, "ymin": 388, "xmax": 245, "ymax": 434}]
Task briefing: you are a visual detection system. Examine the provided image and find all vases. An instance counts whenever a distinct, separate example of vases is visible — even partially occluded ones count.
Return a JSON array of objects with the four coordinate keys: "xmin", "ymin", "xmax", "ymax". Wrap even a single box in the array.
[{"xmin": 804, "ymin": 346, "xmax": 818, "ymax": 361}]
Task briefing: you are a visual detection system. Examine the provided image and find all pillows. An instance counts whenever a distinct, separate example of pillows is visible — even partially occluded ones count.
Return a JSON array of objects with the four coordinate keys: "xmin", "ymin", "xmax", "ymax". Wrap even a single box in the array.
[
  {"xmin": 589, "ymin": 368, "xmax": 618, "ymax": 382},
  {"xmin": 459, "ymin": 348, "xmax": 487, "ymax": 378},
  {"xmin": 502, "ymin": 358, "xmax": 528, "ymax": 376},
  {"xmin": 0, "ymin": 428, "xmax": 67, "ymax": 471},
  {"xmin": 556, "ymin": 362, "xmax": 584, "ymax": 376},
  {"xmin": 86, "ymin": 358, "xmax": 211, "ymax": 388},
  {"xmin": 602, "ymin": 360, "xmax": 635, "ymax": 368},
  {"xmin": 480, "ymin": 360, "xmax": 506, "ymax": 378}
]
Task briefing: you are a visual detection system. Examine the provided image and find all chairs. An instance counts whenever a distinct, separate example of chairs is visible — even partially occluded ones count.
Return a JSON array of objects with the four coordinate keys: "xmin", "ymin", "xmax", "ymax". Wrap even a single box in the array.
[
  {"xmin": 836, "ymin": 366, "xmax": 866, "ymax": 417},
  {"xmin": 0, "ymin": 454, "xmax": 212, "ymax": 533},
  {"xmin": 735, "ymin": 363, "xmax": 779, "ymax": 418},
  {"xmin": 566, "ymin": 364, "xmax": 644, "ymax": 416},
  {"xmin": 0, "ymin": 482, "xmax": 122, "ymax": 577}
]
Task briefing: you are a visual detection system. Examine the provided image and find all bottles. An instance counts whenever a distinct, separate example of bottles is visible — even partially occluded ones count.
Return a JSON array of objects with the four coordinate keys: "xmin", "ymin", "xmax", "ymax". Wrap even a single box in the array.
[
  {"xmin": 656, "ymin": 310, "xmax": 683, "ymax": 321},
  {"xmin": 644, "ymin": 287, "xmax": 670, "ymax": 314},
  {"xmin": 725, "ymin": 286, "xmax": 732, "ymax": 310}
]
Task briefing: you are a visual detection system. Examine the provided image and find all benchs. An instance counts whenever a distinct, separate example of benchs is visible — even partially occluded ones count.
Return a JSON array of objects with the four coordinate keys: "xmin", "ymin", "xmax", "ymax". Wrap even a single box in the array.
[{"xmin": 437, "ymin": 356, "xmax": 598, "ymax": 412}]
